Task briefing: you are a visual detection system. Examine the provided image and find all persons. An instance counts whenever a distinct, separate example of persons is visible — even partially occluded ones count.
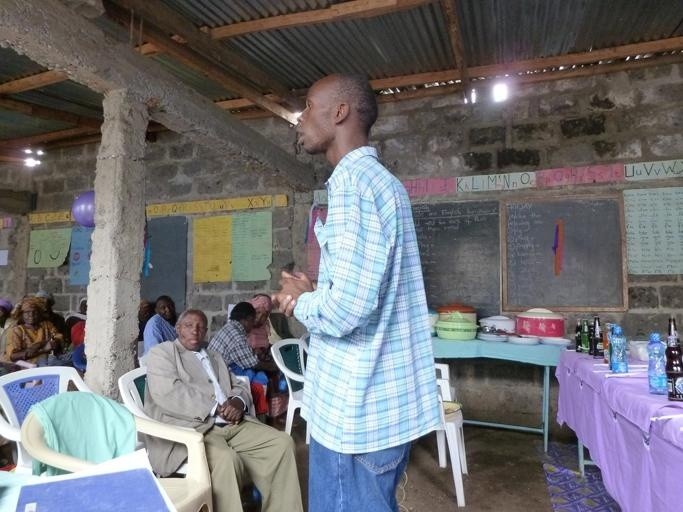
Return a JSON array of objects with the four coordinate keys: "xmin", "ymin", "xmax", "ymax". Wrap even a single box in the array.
[
  {"xmin": 143, "ymin": 294, "xmax": 179, "ymax": 354},
  {"xmin": 205, "ymin": 301, "xmax": 269, "ymax": 386},
  {"xmin": 70, "ymin": 319, "xmax": 85, "ymax": 345},
  {"xmin": 270, "ymin": 70, "xmax": 447, "ymax": 511},
  {"xmin": 244, "ymin": 293, "xmax": 299, "ymax": 393},
  {"xmin": 137, "ymin": 297, "xmax": 157, "ymax": 341},
  {"xmin": 0, "ymin": 288, "xmax": 71, "ymax": 375},
  {"xmin": 137, "ymin": 306, "xmax": 302, "ymax": 511},
  {"xmin": 63, "ymin": 296, "xmax": 88, "ymax": 345}
]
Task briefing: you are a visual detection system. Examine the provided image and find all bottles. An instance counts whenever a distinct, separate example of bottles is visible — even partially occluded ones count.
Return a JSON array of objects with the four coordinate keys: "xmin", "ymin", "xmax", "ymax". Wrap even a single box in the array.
[
  {"xmin": 575, "ymin": 316, "xmax": 683, "ymax": 402},
  {"xmin": 47, "ymin": 335, "xmax": 57, "ymax": 366}
]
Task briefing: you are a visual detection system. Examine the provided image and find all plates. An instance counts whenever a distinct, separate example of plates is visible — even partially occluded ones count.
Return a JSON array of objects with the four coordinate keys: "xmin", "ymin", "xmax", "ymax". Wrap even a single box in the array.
[
  {"xmin": 477, "ymin": 331, "xmax": 572, "ymax": 345},
  {"xmin": 443, "ymin": 401, "xmax": 463, "ymax": 419}
]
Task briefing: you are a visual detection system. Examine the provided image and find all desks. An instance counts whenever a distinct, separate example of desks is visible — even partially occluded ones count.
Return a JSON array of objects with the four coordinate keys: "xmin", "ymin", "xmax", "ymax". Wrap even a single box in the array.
[
  {"xmin": 433, "ymin": 337, "xmax": 565, "ymax": 453},
  {"xmin": 556, "ymin": 349, "xmax": 683, "ymax": 512}
]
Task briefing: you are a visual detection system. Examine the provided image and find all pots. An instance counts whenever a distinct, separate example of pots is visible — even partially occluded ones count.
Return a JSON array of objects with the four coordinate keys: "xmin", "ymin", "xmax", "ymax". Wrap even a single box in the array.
[{"xmin": 428, "ymin": 305, "xmax": 480, "ymax": 341}]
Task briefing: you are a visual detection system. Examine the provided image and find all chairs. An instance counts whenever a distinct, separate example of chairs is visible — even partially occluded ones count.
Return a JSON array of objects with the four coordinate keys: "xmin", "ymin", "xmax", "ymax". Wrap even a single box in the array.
[
  {"xmin": 118, "ymin": 366, "xmax": 256, "ymax": 478},
  {"xmin": 0, "ymin": 366, "xmax": 94, "ymax": 474},
  {"xmin": 439, "ymin": 401, "xmax": 469, "ymax": 509},
  {"xmin": 20, "ymin": 392, "xmax": 213, "ymax": 512},
  {"xmin": 433, "ymin": 363, "xmax": 456, "ymax": 468},
  {"xmin": 271, "ymin": 339, "xmax": 311, "ymax": 445}
]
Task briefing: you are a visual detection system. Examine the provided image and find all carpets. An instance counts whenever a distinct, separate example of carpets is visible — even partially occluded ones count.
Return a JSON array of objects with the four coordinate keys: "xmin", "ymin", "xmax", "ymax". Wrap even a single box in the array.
[{"xmin": 543, "ymin": 462, "xmax": 621, "ymax": 512}]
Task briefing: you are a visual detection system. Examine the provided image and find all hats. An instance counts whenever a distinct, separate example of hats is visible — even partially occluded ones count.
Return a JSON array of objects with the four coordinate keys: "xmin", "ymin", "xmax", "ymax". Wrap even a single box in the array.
[
  {"xmin": 35, "ymin": 290, "xmax": 55, "ymax": 306},
  {"xmin": 0, "ymin": 299, "xmax": 12, "ymax": 313}
]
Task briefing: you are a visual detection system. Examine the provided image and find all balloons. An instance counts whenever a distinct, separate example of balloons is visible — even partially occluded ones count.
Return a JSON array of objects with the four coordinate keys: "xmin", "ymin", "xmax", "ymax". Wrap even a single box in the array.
[{"xmin": 70, "ymin": 190, "xmax": 95, "ymax": 227}]
